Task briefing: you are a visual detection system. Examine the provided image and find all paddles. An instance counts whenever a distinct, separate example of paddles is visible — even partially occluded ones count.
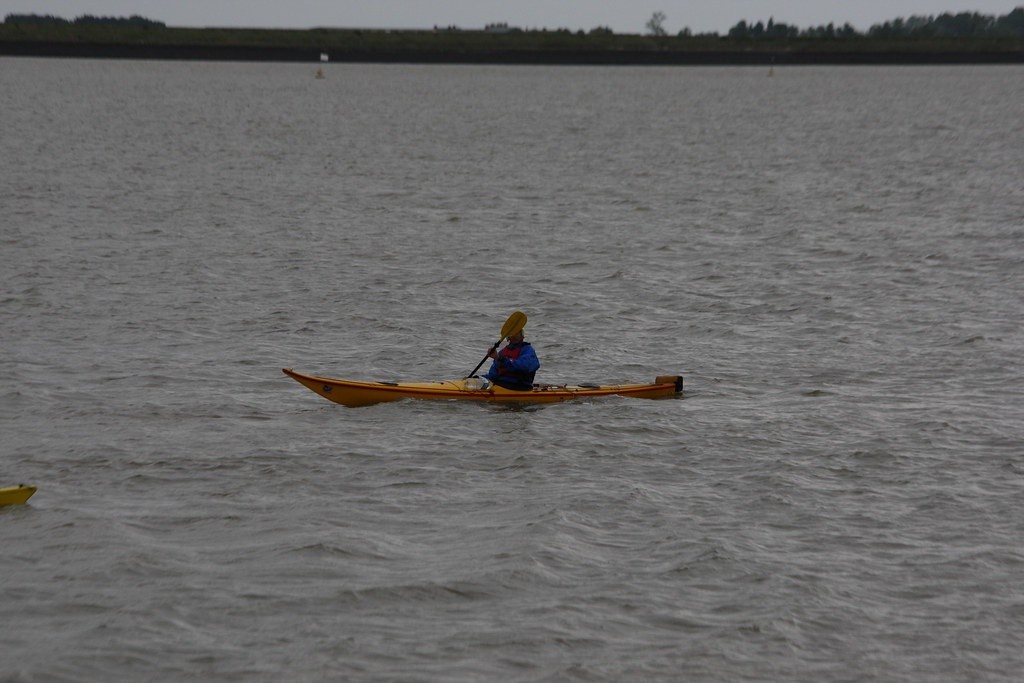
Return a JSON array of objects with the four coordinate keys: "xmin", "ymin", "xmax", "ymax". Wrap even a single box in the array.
[{"xmin": 467, "ymin": 311, "xmax": 528, "ymax": 378}]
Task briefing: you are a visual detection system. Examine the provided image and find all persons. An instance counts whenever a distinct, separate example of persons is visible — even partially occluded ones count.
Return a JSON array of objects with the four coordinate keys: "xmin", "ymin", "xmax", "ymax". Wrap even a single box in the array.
[{"xmin": 480, "ymin": 329, "xmax": 541, "ymax": 391}]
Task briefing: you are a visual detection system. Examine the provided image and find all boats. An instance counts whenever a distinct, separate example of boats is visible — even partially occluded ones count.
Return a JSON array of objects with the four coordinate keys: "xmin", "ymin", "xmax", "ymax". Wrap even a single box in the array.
[
  {"xmin": 282, "ymin": 367, "xmax": 684, "ymax": 409},
  {"xmin": 0, "ymin": 481, "xmax": 38, "ymax": 507}
]
